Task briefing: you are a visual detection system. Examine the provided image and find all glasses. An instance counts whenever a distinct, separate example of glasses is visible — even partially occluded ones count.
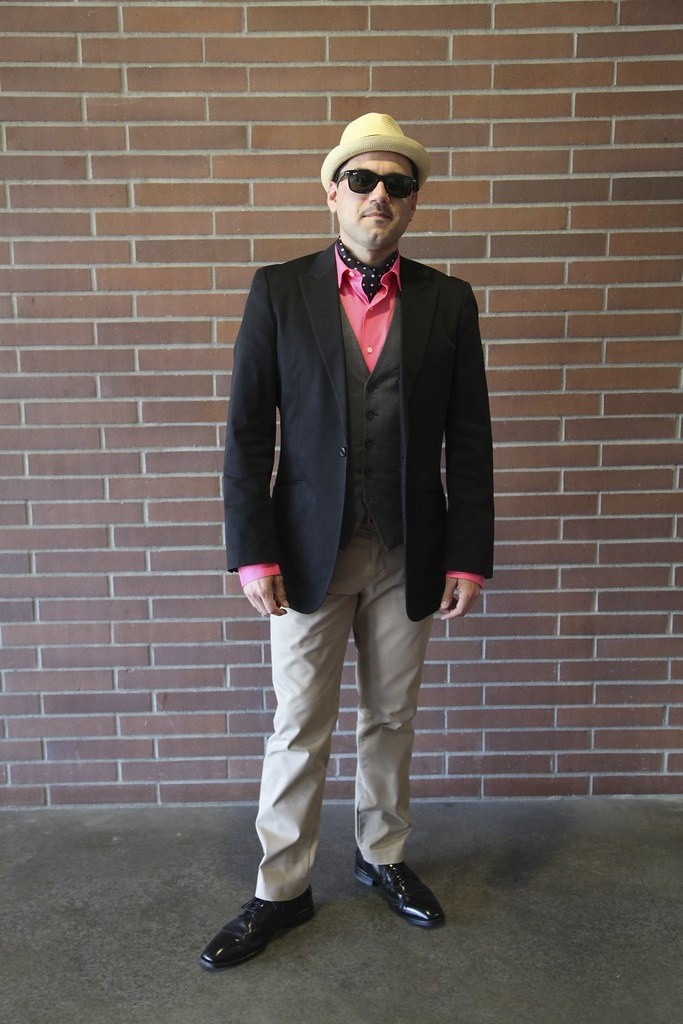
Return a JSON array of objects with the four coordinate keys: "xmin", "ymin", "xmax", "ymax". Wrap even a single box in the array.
[{"xmin": 337, "ymin": 170, "xmax": 417, "ymax": 198}]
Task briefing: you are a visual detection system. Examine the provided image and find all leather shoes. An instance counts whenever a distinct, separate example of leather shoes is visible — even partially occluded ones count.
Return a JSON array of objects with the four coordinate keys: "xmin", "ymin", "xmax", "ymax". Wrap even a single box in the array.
[
  {"xmin": 200, "ymin": 883, "xmax": 314, "ymax": 969},
  {"xmin": 355, "ymin": 848, "xmax": 445, "ymax": 928}
]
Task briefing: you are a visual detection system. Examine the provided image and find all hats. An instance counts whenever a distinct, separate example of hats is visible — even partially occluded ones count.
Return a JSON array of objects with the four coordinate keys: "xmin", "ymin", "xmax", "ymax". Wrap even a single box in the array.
[{"xmin": 321, "ymin": 112, "xmax": 431, "ymax": 193}]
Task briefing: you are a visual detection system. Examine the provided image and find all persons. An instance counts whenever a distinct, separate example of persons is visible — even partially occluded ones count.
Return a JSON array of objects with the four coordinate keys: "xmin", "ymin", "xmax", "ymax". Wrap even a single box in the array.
[{"xmin": 200, "ymin": 111, "xmax": 495, "ymax": 971}]
